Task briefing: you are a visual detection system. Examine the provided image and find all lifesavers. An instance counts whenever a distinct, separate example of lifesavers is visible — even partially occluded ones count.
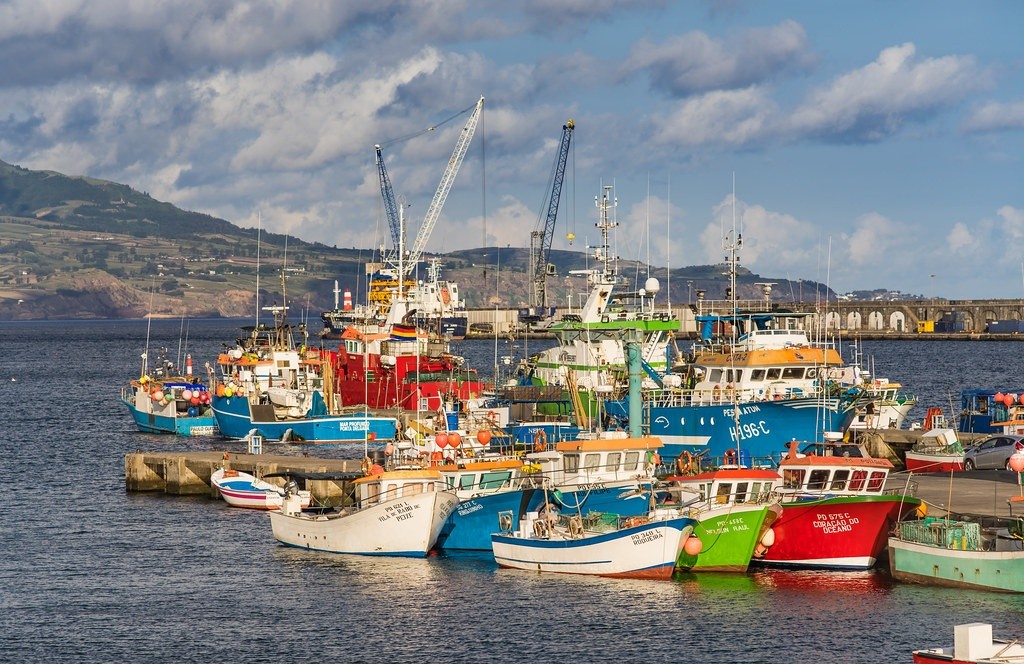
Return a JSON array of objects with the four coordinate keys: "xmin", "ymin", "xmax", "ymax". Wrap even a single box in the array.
[
  {"xmin": 725, "ymin": 385, "xmax": 733, "ymax": 400},
  {"xmin": 678, "ymin": 450, "xmax": 692, "ymax": 474},
  {"xmin": 625, "ymin": 517, "xmax": 648, "ymax": 526},
  {"xmin": 361, "ymin": 456, "xmax": 373, "ymax": 476},
  {"xmin": 535, "ymin": 429, "xmax": 547, "ymax": 451},
  {"xmin": 723, "ymin": 450, "xmax": 736, "ymax": 465},
  {"xmin": 713, "ymin": 385, "xmax": 721, "ymax": 401},
  {"xmin": 487, "ymin": 410, "xmax": 497, "ymax": 427},
  {"xmin": 352, "ymin": 371, "xmax": 357, "ymax": 380}
]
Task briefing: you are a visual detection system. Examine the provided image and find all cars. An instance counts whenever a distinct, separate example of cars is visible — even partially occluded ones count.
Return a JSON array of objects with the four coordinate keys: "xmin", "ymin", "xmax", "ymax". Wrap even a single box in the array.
[{"xmin": 962, "ymin": 434, "xmax": 1024, "ymax": 471}]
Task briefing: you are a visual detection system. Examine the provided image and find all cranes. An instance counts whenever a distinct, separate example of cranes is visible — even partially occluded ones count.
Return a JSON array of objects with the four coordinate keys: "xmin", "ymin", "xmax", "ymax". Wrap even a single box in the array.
[{"xmin": 517, "ymin": 118, "xmax": 575, "ymax": 324}]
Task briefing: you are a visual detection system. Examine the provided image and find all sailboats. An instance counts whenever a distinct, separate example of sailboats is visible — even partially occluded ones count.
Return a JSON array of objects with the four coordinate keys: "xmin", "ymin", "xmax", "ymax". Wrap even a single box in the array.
[{"xmin": 121, "ymin": 204, "xmax": 1023, "ymax": 594}]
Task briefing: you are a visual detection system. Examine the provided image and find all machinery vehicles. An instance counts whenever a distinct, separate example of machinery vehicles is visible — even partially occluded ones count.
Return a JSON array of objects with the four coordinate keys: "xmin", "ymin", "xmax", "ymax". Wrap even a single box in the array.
[
  {"xmin": 402, "ymin": 95, "xmax": 494, "ymax": 288},
  {"xmin": 373, "ymin": 144, "xmax": 401, "ymax": 281}
]
